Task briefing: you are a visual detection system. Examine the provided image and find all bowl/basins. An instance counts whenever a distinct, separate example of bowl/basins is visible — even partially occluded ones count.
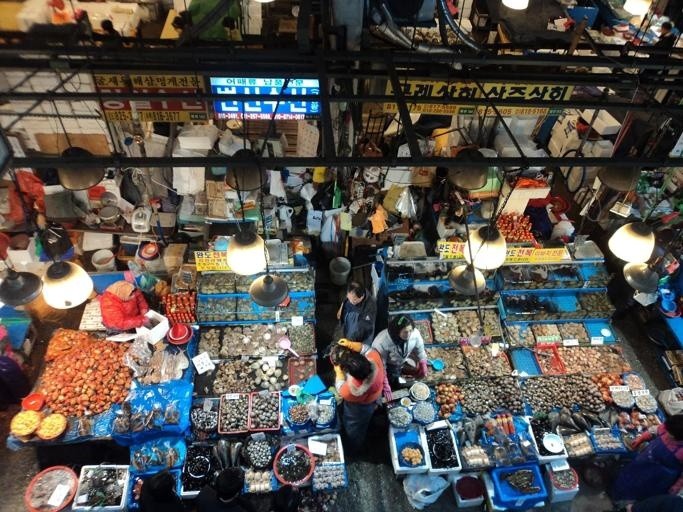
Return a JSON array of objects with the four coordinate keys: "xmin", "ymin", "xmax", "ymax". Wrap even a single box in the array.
[
  {"xmin": 22, "ymin": 394, "xmax": 44, "ymax": 410},
  {"xmin": 273, "ymin": 443, "xmax": 315, "ymax": 486},
  {"xmin": 399, "ymin": 441, "xmax": 425, "ymax": 467}
]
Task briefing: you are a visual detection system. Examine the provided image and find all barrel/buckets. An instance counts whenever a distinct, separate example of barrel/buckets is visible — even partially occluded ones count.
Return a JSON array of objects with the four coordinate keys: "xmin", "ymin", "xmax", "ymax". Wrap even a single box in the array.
[
  {"xmin": 91, "ymin": 249, "xmax": 116, "ymax": 272},
  {"xmin": 329, "ymin": 256, "xmax": 352, "ymax": 285}
]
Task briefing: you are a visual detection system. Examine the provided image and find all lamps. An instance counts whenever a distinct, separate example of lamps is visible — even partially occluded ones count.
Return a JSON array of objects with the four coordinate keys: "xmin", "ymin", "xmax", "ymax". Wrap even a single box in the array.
[
  {"xmin": 622, "ymin": 0, "xmax": 653, "ymax": 17},
  {"xmin": 502, "ymin": 0, "xmax": 530, "ymax": 11},
  {"xmin": 247, "ymin": 180, "xmax": 291, "ymax": 307},
  {"xmin": 445, "ymin": 174, "xmax": 524, "ymax": 297},
  {"xmin": 223, "ymin": 101, "xmax": 268, "ymax": 192},
  {"xmin": 624, "ymin": 229, "xmax": 682, "ymax": 296},
  {"xmin": 448, "ymin": 144, "xmax": 490, "ymax": 193},
  {"xmin": 462, "ymin": 167, "xmax": 528, "ymax": 272},
  {"xmin": 600, "ymin": 157, "xmax": 641, "ymax": 191},
  {"xmin": 43, "ymin": 92, "xmax": 107, "ymax": 192},
  {"xmin": 224, "ymin": 155, "xmax": 271, "ymax": 277},
  {"xmin": 1, "ymin": 149, "xmax": 94, "ymax": 310},
  {"xmin": 606, "ymin": 186, "xmax": 676, "ymax": 261}
]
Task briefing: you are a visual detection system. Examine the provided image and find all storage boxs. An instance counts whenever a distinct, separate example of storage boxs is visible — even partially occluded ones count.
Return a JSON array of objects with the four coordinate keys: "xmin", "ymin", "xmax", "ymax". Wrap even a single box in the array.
[{"xmin": 0, "ymin": 179, "xmax": 683, "ymax": 512}]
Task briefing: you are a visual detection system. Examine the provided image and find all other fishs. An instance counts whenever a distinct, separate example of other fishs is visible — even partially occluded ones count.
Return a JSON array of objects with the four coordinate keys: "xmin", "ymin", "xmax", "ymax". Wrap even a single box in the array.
[
  {"xmin": 211, "ymin": 437, "xmax": 243, "ymax": 472},
  {"xmin": 454, "ymin": 414, "xmax": 484, "ymax": 449},
  {"xmin": 547, "ymin": 406, "xmax": 619, "ymax": 436}
]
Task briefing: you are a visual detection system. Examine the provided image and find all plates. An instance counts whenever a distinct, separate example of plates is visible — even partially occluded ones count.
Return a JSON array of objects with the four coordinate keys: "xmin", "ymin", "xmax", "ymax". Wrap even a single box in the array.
[{"xmin": 542, "ymin": 432, "xmax": 563, "ymax": 454}]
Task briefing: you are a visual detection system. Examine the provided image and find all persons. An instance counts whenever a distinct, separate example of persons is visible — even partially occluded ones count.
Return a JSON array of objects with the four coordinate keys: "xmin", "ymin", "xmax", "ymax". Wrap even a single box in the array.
[
  {"xmin": 100, "ymin": 280, "xmax": 153, "ymax": 336},
  {"xmin": 640, "ymin": 23, "xmax": 678, "ymax": 84},
  {"xmin": 600, "ymin": 414, "xmax": 683, "ymax": 512},
  {"xmin": 327, "ymin": 280, "xmax": 428, "ymax": 451},
  {"xmin": 73, "ymin": 8, "xmax": 250, "ymax": 48}
]
